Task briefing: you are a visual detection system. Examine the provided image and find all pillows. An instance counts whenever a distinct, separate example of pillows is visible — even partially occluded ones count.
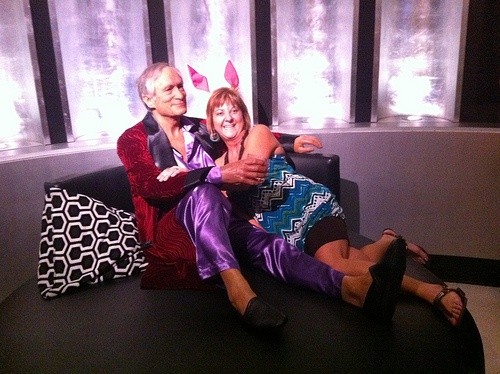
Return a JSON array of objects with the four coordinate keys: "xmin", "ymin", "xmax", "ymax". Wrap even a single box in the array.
[{"xmin": 36, "ymin": 186, "xmax": 150, "ymax": 299}]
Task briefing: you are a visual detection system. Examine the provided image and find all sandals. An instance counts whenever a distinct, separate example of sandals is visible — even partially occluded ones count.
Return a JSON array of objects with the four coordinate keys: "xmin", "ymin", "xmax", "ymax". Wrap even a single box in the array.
[
  {"xmin": 430, "ymin": 282, "xmax": 468, "ymax": 327},
  {"xmin": 381, "ymin": 227, "xmax": 431, "ymax": 267}
]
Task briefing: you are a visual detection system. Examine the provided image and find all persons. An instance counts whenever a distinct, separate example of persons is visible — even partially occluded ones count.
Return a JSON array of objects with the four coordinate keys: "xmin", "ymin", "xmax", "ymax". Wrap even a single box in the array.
[
  {"xmin": 117, "ymin": 64, "xmax": 407, "ymax": 331},
  {"xmin": 207, "ymin": 88, "xmax": 466, "ymax": 328}
]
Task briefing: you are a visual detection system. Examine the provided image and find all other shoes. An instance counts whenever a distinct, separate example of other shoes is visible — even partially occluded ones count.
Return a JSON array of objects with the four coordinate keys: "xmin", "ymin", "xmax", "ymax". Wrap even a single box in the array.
[
  {"xmin": 231, "ymin": 295, "xmax": 289, "ymax": 335},
  {"xmin": 364, "ymin": 238, "xmax": 408, "ymax": 325}
]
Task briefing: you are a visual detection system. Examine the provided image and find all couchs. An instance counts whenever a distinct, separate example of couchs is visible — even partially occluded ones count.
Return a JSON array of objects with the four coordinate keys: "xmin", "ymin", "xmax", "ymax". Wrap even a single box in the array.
[{"xmin": 0, "ymin": 153, "xmax": 485, "ymax": 374}]
